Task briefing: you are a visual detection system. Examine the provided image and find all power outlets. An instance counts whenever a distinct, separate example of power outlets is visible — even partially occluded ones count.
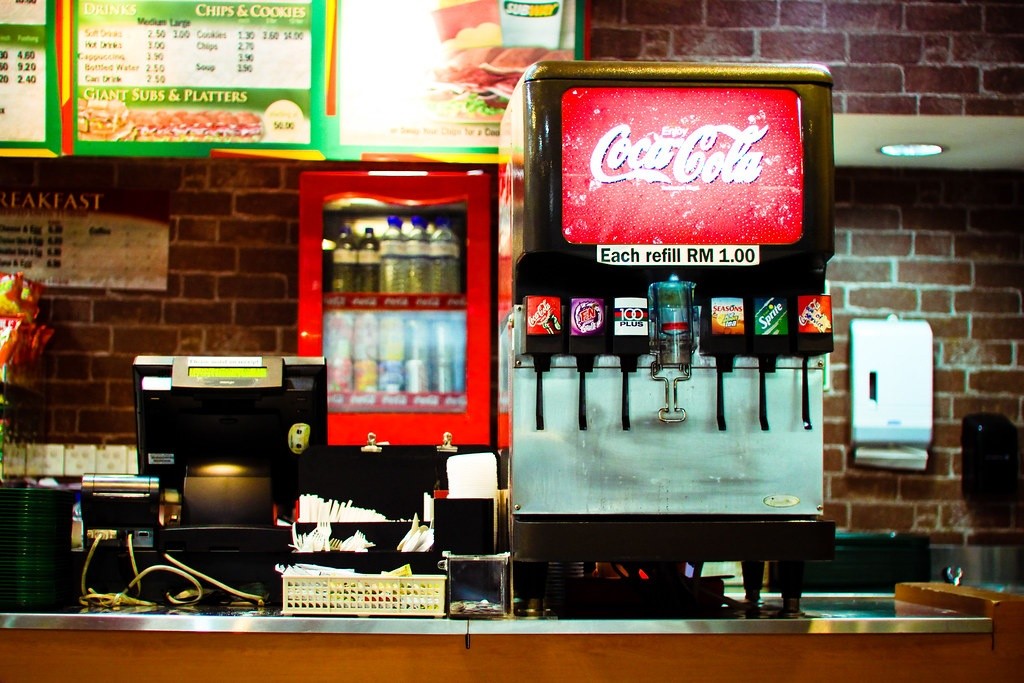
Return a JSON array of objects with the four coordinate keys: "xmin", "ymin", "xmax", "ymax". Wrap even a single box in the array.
[{"xmin": 2, "ymin": 443, "xmax": 138, "ymax": 476}]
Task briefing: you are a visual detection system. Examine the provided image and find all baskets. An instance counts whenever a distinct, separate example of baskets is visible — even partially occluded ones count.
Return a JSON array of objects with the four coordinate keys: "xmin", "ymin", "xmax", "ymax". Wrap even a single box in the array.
[{"xmin": 281, "ymin": 574, "xmax": 447, "ymax": 617}]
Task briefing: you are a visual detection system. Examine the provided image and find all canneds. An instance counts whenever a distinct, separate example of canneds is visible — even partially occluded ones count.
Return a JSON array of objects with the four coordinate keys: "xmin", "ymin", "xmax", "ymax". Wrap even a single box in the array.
[{"xmin": 324, "ymin": 310, "xmax": 466, "ymax": 397}]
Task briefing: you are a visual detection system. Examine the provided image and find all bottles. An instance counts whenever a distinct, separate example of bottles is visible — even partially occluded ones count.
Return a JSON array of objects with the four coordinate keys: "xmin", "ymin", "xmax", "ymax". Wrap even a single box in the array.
[{"xmin": 330, "ymin": 215, "xmax": 466, "ymax": 294}]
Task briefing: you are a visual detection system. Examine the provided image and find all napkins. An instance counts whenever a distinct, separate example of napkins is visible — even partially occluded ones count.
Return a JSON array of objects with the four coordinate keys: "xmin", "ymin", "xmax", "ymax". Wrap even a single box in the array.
[{"xmin": 285, "ymin": 492, "xmax": 434, "ymax": 523}]
